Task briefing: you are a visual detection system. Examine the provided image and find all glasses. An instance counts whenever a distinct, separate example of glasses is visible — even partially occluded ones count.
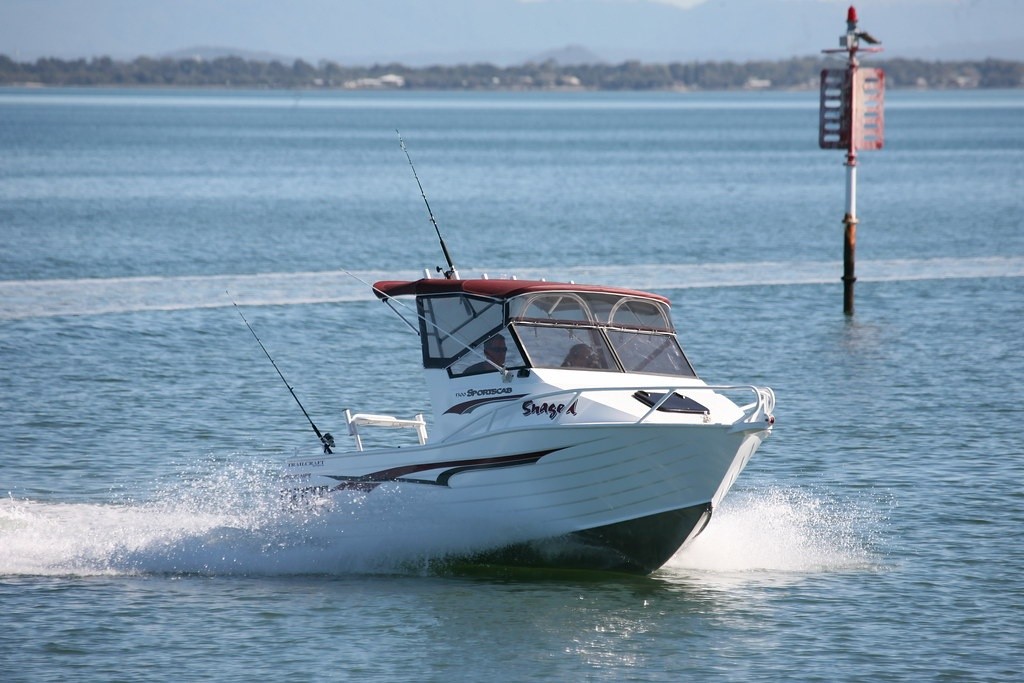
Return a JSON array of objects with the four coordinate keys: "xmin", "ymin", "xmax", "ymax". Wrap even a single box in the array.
[{"xmin": 487, "ymin": 346, "xmax": 507, "ymax": 352}]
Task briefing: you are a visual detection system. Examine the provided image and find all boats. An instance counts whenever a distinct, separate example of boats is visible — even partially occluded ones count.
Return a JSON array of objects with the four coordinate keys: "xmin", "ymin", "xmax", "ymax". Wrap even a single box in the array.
[{"xmin": 113, "ymin": 127, "xmax": 776, "ymax": 577}]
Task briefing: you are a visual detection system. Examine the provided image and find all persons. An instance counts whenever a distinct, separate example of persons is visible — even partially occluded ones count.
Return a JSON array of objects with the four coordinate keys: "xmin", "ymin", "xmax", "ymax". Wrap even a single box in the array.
[
  {"xmin": 462, "ymin": 334, "xmax": 507, "ymax": 373},
  {"xmin": 561, "ymin": 344, "xmax": 600, "ymax": 368}
]
